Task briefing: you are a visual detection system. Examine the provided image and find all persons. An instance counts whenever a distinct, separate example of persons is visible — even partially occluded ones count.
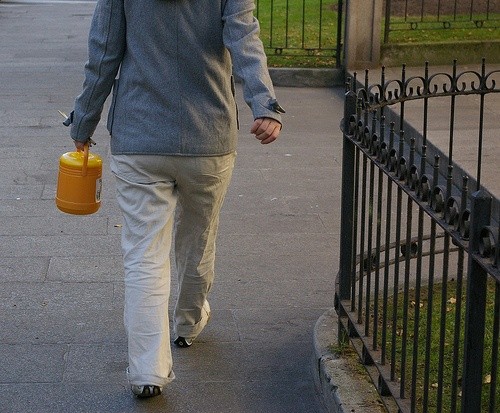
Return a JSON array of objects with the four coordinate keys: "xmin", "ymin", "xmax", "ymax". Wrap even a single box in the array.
[{"xmin": 63, "ymin": 0, "xmax": 285, "ymax": 398}]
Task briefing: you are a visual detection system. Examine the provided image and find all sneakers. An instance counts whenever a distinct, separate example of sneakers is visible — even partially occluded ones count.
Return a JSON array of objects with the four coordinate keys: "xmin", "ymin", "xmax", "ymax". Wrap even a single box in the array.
[
  {"xmin": 131, "ymin": 385, "xmax": 161, "ymax": 397},
  {"xmin": 173, "ymin": 299, "xmax": 210, "ymax": 348}
]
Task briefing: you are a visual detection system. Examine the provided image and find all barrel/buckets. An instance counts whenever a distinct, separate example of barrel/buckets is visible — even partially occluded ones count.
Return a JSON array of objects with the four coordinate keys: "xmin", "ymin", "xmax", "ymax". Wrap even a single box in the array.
[{"xmin": 55, "ymin": 143, "xmax": 103, "ymax": 215}]
